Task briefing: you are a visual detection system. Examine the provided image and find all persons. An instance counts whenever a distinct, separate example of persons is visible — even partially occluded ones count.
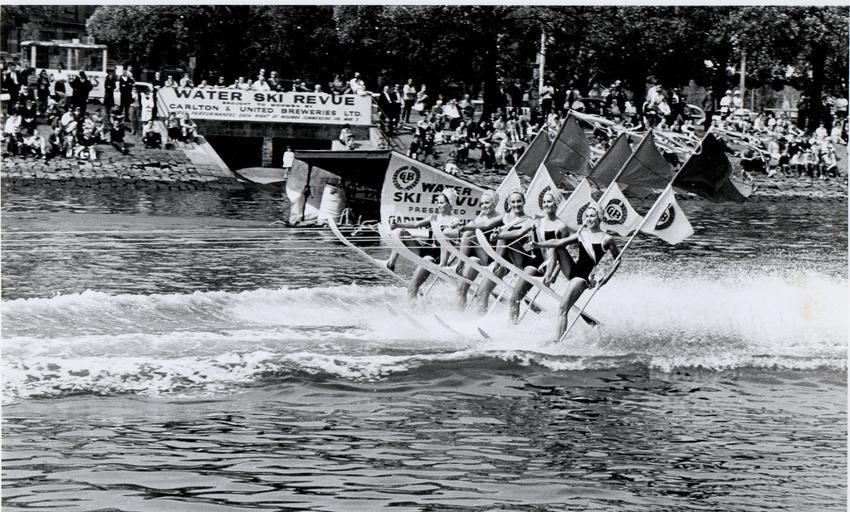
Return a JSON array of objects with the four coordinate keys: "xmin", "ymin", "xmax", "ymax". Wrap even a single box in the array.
[
  {"xmin": 0, "ymin": 56, "xmax": 849, "ymax": 184},
  {"xmin": 374, "ymin": 188, "xmax": 622, "ymax": 348}
]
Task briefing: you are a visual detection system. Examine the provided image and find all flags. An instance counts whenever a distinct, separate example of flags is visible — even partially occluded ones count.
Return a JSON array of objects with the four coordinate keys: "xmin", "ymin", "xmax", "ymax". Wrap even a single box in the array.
[{"xmin": 376, "ymin": 109, "xmax": 760, "ymax": 249}]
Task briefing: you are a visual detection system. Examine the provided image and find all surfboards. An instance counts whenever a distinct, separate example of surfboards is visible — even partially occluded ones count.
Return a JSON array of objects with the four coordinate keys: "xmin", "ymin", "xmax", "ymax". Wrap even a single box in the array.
[{"xmin": 326, "ymin": 214, "xmax": 603, "ymax": 338}]
